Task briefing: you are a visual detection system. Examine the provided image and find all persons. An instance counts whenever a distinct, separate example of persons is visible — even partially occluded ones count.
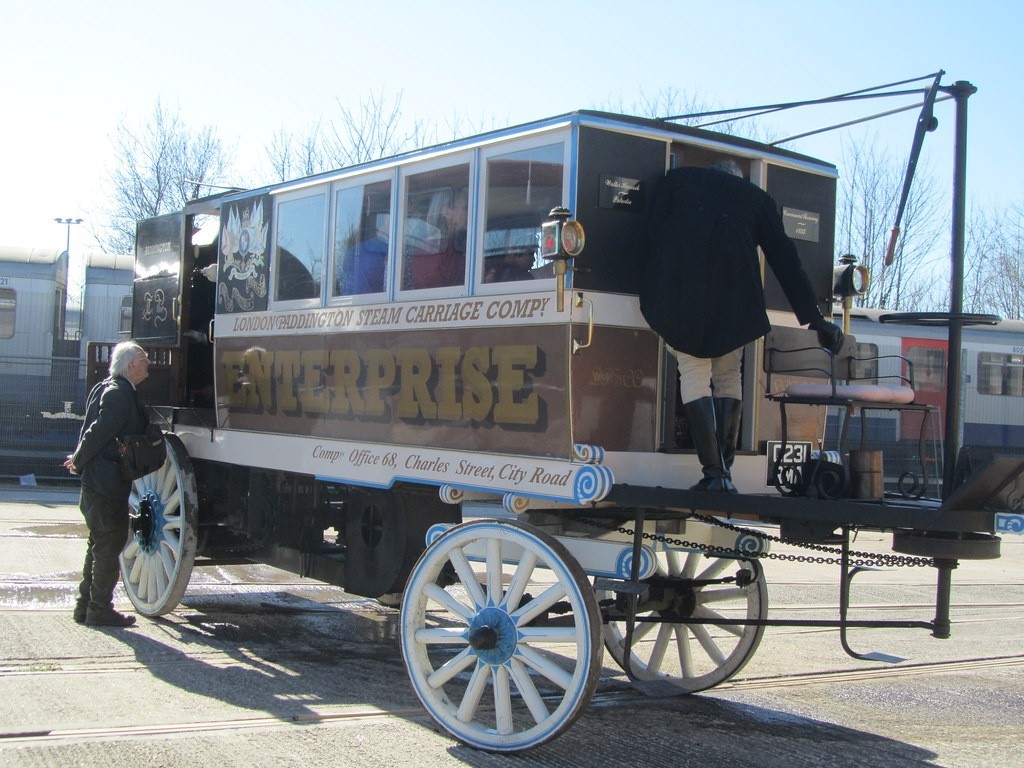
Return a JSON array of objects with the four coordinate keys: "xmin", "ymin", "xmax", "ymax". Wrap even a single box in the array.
[
  {"xmin": 64, "ymin": 341, "xmax": 150, "ymax": 627},
  {"xmin": 406, "ymin": 187, "xmax": 495, "ymax": 288},
  {"xmin": 340, "ymin": 196, "xmax": 426, "ymax": 295},
  {"xmin": 635, "ymin": 160, "xmax": 844, "ymax": 490}
]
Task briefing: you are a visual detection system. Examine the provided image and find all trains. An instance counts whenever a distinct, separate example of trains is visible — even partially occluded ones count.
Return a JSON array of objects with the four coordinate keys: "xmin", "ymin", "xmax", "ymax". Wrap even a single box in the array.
[{"xmin": 0, "ymin": 243, "xmax": 1024, "ymax": 481}]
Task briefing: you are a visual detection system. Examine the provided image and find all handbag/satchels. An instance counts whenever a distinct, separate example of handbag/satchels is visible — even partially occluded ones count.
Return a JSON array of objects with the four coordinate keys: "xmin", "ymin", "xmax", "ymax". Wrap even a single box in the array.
[{"xmin": 119, "ymin": 425, "xmax": 166, "ymax": 480}]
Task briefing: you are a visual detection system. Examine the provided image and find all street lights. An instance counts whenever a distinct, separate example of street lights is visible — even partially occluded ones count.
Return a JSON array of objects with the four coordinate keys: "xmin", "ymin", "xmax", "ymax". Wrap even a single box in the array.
[{"xmin": 54, "ymin": 216, "xmax": 84, "ymax": 341}]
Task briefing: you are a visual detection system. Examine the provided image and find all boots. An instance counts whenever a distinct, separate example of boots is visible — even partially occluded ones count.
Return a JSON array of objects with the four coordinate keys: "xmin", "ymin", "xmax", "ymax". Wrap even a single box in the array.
[
  {"xmin": 683, "ymin": 396, "xmax": 725, "ymax": 492},
  {"xmin": 714, "ymin": 397, "xmax": 744, "ymax": 493}
]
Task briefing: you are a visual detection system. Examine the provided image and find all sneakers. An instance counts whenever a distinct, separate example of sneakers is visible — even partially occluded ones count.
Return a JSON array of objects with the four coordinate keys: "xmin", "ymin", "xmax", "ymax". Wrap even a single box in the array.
[
  {"xmin": 74, "ymin": 607, "xmax": 87, "ymax": 621},
  {"xmin": 86, "ymin": 609, "xmax": 136, "ymax": 626}
]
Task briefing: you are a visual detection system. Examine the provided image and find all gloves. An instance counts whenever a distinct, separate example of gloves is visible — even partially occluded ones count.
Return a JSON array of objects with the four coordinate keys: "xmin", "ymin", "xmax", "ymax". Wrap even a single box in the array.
[{"xmin": 808, "ymin": 317, "xmax": 845, "ymax": 354}]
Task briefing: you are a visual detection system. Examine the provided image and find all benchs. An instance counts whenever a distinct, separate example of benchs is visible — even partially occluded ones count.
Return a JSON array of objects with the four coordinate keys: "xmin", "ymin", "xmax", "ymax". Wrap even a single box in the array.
[{"xmin": 762, "ymin": 325, "xmax": 940, "ymax": 501}]
